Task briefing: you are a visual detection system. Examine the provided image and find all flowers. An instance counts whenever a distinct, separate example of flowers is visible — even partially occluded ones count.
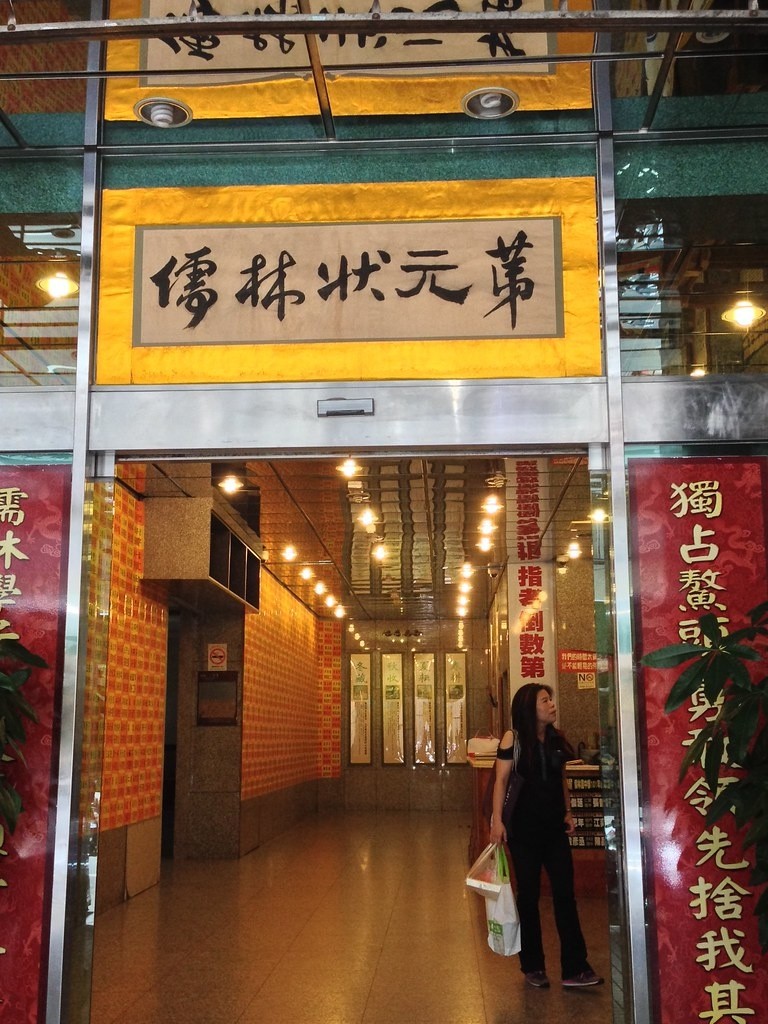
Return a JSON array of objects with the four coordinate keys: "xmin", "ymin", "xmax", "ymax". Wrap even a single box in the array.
[{"xmin": 443, "ymin": 651, "xmax": 470, "ymax": 767}]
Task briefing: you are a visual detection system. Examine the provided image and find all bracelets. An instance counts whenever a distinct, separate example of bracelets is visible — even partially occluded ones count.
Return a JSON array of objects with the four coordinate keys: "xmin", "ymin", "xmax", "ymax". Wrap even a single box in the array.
[{"xmin": 567, "ymin": 808, "xmax": 571, "ymax": 811}]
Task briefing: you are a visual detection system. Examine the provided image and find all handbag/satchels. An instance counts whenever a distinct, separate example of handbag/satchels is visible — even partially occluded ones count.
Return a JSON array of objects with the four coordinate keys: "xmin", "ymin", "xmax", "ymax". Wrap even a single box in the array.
[
  {"xmin": 467, "ymin": 727, "xmax": 501, "ymax": 754},
  {"xmin": 485, "ymin": 843, "xmax": 522, "ymax": 956},
  {"xmin": 481, "ymin": 760, "xmax": 525, "ymax": 826}
]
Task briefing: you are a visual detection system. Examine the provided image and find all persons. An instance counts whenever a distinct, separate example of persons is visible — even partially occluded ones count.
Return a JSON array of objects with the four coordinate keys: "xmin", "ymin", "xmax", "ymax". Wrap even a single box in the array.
[{"xmin": 489, "ymin": 683, "xmax": 605, "ymax": 987}]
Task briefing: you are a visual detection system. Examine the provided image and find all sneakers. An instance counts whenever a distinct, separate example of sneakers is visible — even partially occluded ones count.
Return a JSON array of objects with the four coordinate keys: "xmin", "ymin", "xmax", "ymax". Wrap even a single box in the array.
[
  {"xmin": 524, "ymin": 969, "xmax": 550, "ymax": 987},
  {"xmin": 560, "ymin": 974, "xmax": 605, "ymax": 987}
]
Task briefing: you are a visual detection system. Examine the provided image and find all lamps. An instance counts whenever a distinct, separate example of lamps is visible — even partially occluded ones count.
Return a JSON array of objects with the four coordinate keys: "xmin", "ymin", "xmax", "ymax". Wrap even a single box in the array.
[
  {"xmin": 150, "ymin": 102, "xmax": 174, "ymax": 128},
  {"xmin": 479, "ymin": 92, "xmax": 503, "ymax": 109}
]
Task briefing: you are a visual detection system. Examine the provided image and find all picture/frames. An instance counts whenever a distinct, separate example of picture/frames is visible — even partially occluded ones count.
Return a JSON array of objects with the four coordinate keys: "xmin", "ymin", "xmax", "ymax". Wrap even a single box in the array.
[
  {"xmin": 348, "ymin": 651, "xmax": 373, "ymax": 767},
  {"xmin": 380, "ymin": 650, "xmax": 406, "ymax": 767},
  {"xmin": 412, "ymin": 651, "xmax": 438, "ymax": 766}
]
